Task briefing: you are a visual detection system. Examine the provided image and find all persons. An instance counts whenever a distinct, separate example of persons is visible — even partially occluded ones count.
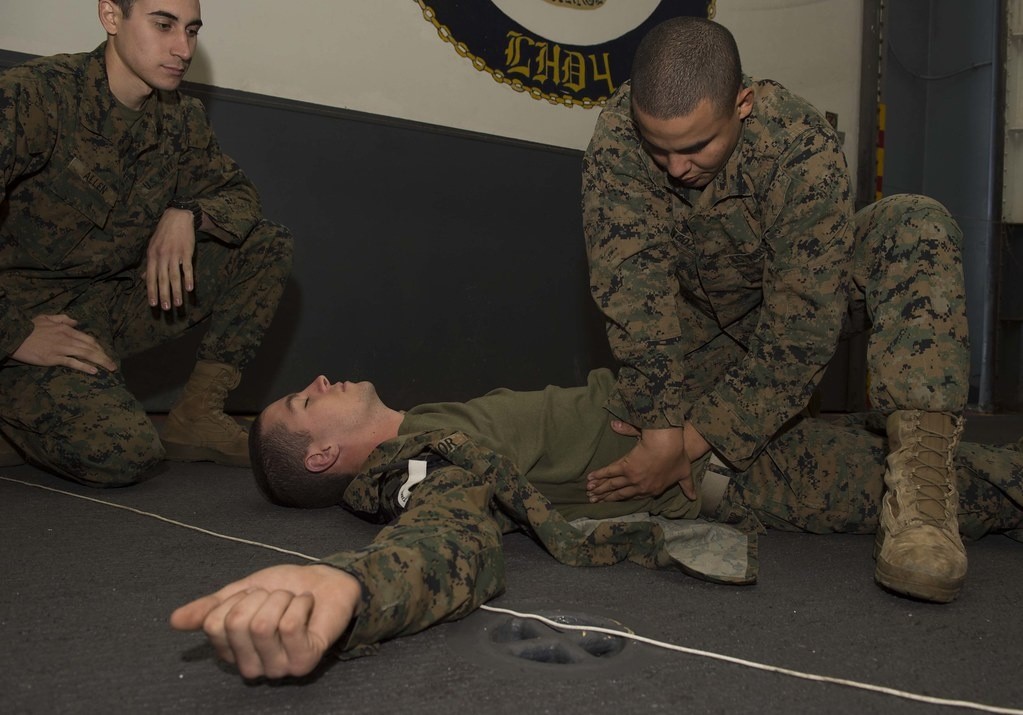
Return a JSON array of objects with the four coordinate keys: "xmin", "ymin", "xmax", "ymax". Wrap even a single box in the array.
[
  {"xmin": 580, "ymin": 16, "xmax": 971, "ymax": 604},
  {"xmin": 0, "ymin": 0, "xmax": 296, "ymax": 489},
  {"xmin": 169, "ymin": 367, "xmax": 1023, "ymax": 680}
]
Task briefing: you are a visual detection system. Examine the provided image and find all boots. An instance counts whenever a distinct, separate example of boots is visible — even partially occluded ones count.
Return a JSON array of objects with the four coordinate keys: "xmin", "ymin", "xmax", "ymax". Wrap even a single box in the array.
[
  {"xmin": 875, "ymin": 409, "xmax": 971, "ymax": 603},
  {"xmin": 159, "ymin": 360, "xmax": 252, "ymax": 467}
]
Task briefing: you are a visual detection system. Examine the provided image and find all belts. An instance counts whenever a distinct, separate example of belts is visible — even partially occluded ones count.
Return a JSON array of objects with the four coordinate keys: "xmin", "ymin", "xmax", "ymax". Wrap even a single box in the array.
[{"xmin": 698, "ymin": 451, "xmax": 731, "ymax": 518}]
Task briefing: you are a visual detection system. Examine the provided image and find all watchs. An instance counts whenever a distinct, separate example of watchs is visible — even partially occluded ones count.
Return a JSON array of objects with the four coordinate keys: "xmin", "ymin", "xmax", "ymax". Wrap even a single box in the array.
[{"xmin": 166, "ymin": 194, "xmax": 203, "ymax": 230}]
